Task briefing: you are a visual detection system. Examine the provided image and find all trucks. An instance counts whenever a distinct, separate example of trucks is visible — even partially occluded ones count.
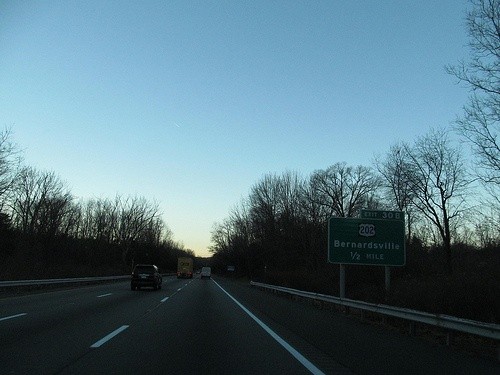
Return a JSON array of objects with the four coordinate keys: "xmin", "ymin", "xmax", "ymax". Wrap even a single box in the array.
[{"xmin": 176, "ymin": 257, "xmax": 193, "ymax": 278}]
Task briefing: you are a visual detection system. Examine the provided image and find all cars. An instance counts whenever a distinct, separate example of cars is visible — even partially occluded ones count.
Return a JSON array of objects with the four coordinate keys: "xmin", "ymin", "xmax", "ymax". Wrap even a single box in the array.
[
  {"xmin": 201, "ymin": 267, "xmax": 211, "ymax": 279},
  {"xmin": 130, "ymin": 264, "xmax": 163, "ymax": 290}
]
